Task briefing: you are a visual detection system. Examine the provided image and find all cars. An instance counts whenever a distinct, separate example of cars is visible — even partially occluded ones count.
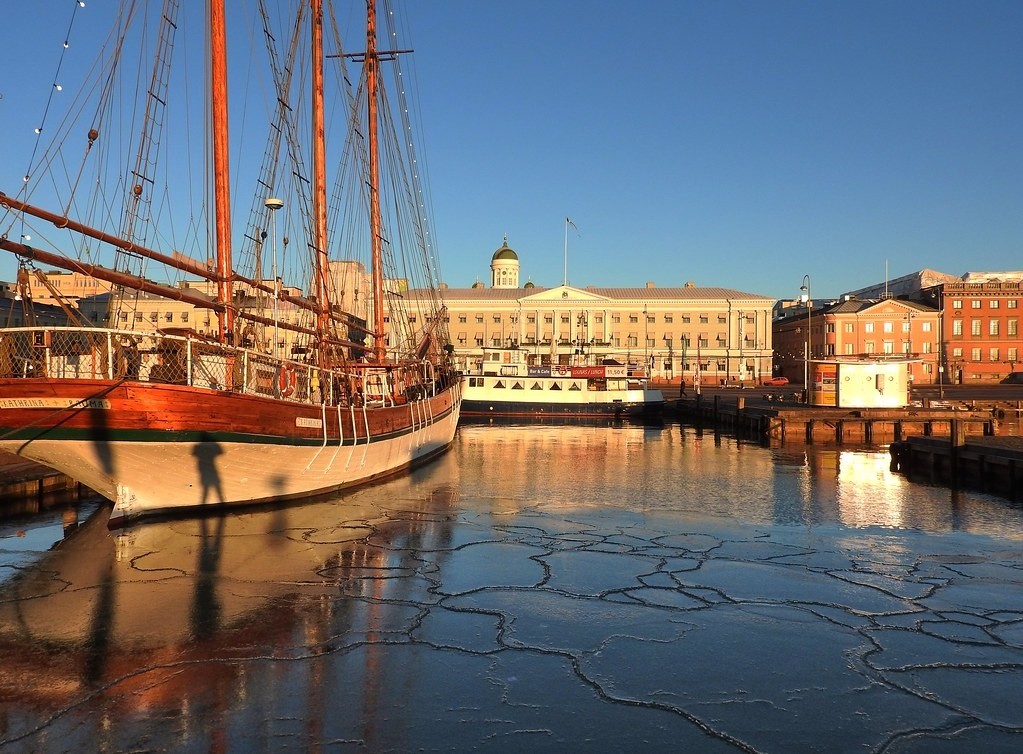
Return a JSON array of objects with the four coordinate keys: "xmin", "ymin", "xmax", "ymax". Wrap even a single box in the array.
[
  {"xmin": 764, "ymin": 377, "xmax": 789, "ymax": 387},
  {"xmin": 1000, "ymin": 372, "xmax": 1023, "ymax": 384}
]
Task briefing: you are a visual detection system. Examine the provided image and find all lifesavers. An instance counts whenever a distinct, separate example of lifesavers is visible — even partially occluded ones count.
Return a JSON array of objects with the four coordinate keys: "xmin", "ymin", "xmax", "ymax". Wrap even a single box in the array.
[
  {"xmin": 558, "ymin": 367, "xmax": 567, "ymax": 375},
  {"xmin": 968, "ymin": 406, "xmax": 979, "ymax": 412},
  {"xmin": 993, "ymin": 407, "xmax": 1007, "ymax": 421},
  {"xmin": 279, "ymin": 361, "xmax": 296, "ymax": 396}
]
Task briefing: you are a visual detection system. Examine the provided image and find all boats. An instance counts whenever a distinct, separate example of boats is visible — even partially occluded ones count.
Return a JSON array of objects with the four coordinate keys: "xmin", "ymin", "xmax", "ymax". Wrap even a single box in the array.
[
  {"xmin": 458, "ymin": 335, "xmax": 668, "ymax": 415},
  {"xmin": 0, "ymin": 1, "xmax": 466, "ymax": 532}
]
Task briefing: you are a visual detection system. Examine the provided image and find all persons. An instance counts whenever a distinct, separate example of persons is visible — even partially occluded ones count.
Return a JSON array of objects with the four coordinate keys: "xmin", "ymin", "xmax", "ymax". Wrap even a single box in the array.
[
  {"xmin": 732, "ymin": 376, "xmax": 735, "ymax": 380},
  {"xmin": 680, "ymin": 379, "xmax": 687, "ymax": 398},
  {"xmin": 720, "ymin": 378, "xmax": 726, "ymax": 390}
]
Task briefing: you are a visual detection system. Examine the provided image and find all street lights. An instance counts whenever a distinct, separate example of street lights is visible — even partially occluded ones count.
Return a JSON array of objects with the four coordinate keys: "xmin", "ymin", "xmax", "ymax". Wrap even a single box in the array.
[
  {"xmin": 800, "ymin": 275, "xmax": 811, "ymax": 360},
  {"xmin": 930, "ymin": 286, "xmax": 943, "ymax": 399}
]
